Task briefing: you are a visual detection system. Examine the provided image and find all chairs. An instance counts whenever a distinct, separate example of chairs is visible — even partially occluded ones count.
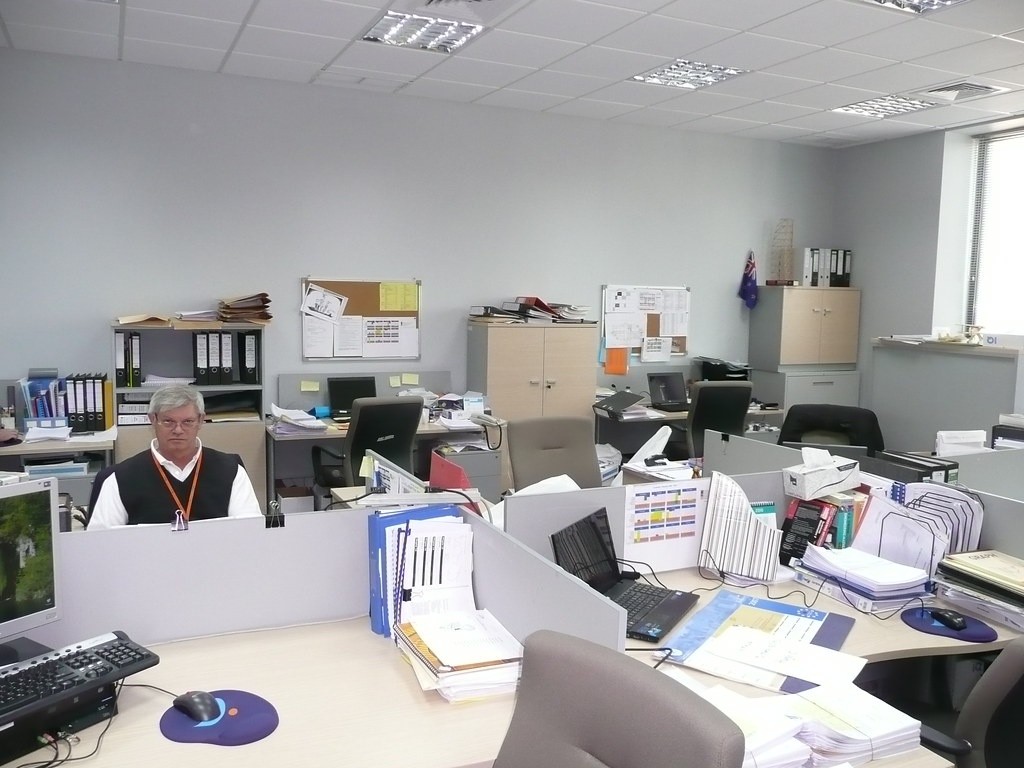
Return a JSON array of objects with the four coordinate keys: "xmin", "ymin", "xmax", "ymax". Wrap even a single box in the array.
[
  {"xmin": 313, "ymin": 396, "xmax": 424, "ymax": 488},
  {"xmin": 919, "ymin": 633, "xmax": 1024, "ymax": 768},
  {"xmin": 660, "ymin": 381, "xmax": 753, "ymax": 461},
  {"xmin": 776, "ymin": 404, "xmax": 884, "ymax": 458},
  {"xmin": 507, "ymin": 417, "xmax": 603, "ymax": 493},
  {"xmin": 493, "ymin": 630, "xmax": 744, "ymax": 768}
]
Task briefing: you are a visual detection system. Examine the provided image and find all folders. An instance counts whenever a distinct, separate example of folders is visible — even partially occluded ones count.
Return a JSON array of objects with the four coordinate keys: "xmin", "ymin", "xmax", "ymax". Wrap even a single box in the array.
[
  {"xmin": 860, "ymin": 449, "xmax": 959, "ymax": 484},
  {"xmin": 501, "ymin": 302, "xmax": 557, "ymax": 320},
  {"xmin": 116, "ymin": 332, "xmax": 127, "ymax": 388},
  {"xmin": 75, "ymin": 373, "xmax": 86, "ymax": 433},
  {"xmin": 117, "ymin": 393, "xmax": 153, "ymax": 426},
  {"xmin": 65, "ymin": 373, "xmax": 79, "ymax": 433},
  {"xmin": 85, "ymin": 373, "xmax": 97, "ymax": 431},
  {"xmin": 130, "ymin": 331, "xmax": 141, "ymax": 387},
  {"xmin": 515, "ymin": 296, "xmax": 560, "ymax": 317},
  {"xmin": 368, "ymin": 503, "xmax": 460, "ymax": 638},
  {"xmin": 469, "ymin": 305, "xmax": 525, "ymax": 319},
  {"xmin": 593, "ymin": 390, "xmax": 651, "ymax": 423},
  {"xmin": 220, "ymin": 331, "xmax": 232, "ymax": 385},
  {"xmin": 208, "ymin": 331, "xmax": 220, "ymax": 385},
  {"xmin": 93, "ymin": 372, "xmax": 108, "ymax": 432},
  {"xmin": 191, "ymin": 331, "xmax": 208, "ymax": 385},
  {"xmin": 778, "ymin": 247, "xmax": 851, "ymax": 287},
  {"xmin": 794, "ymin": 571, "xmax": 936, "ymax": 614},
  {"xmin": 237, "ymin": 332, "xmax": 256, "ymax": 384}
]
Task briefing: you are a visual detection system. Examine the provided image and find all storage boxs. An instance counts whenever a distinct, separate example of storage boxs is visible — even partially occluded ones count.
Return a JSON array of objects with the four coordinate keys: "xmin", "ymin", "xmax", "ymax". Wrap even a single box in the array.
[{"xmin": 275, "ymin": 486, "xmax": 343, "ymax": 515}]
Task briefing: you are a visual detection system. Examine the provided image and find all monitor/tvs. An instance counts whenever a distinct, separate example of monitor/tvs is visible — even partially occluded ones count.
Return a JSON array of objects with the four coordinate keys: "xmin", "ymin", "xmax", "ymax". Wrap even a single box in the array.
[
  {"xmin": 0, "ymin": 477, "xmax": 62, "ymax": 645},
  {"xmin": 782, "ymin": 442, "xmax": 868, "ymax": 459}
]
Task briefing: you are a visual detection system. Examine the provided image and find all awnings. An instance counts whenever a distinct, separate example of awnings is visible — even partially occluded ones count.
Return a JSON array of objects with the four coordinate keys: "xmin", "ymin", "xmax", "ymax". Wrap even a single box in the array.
[{"xmin": 934, "ymin": 413, "xmax": 1024, "ymax": 457}]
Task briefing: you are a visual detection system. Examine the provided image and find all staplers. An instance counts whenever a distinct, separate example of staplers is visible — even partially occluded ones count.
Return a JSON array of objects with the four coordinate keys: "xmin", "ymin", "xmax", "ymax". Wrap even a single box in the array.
[{"xmin": 644, "ymin": 454, "xmax": 667, "ymax": 467}]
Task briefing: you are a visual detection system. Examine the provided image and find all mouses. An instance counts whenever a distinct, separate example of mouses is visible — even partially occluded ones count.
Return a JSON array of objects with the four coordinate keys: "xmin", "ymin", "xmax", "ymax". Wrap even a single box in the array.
[
  {"xmin": 172, "ymin": 691, "xmax": 222, "ymax": 721},
  {"xmin": 930, "ymin": 610, "xmax": 966, "ymax": 631}
]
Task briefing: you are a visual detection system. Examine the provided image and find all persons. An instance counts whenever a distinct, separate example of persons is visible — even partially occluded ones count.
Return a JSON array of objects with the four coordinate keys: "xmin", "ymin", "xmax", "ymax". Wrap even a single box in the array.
[
  {"xmin": 83, "ymin": 384, "xmax": 265, "ymax": 531},
  {"xmin": 0, "ymin": 428, "xmax": 19, "ymax": 443}
]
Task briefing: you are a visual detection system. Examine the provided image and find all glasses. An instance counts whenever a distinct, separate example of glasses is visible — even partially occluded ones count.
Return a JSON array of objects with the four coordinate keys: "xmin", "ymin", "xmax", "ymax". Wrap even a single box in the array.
[{"xmin": 155, "ymin": 412, "xmax": 201, "ymax": 428}]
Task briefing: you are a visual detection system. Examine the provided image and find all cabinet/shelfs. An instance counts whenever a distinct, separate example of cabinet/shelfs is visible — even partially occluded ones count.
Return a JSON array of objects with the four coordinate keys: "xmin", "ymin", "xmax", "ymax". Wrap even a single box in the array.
[
  {"xmin": 748, "ymin": 369, "xmax": 862, "ymax": 423},
  {"xmin": 746, "ymin": 284, "xmax": 860, "ymax": 374},
  {"xmin": 53, "ymin": 460, "xmax": 104, "ymax": 510},
  {"xmin": 111, "ymin": 321, "xmax": 269, "ymax": 517},
  {"xmin": 435, "ymin": 449, "xmax": 502, "ymax": 506},
  {"xmin": 466, "ymin": 320, "xmax": 598, "ymax": 490}
]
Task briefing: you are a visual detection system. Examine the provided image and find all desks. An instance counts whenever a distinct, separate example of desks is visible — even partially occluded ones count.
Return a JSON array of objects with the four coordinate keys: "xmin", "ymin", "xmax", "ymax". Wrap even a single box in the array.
[
  {"xmin": 626, "ymin": 563, "xmax": 1024, "ymax": 768},
  {"xmin": 265, "ymin": 419, "xmax": 485, "ymax": 515},
  {"xmin": 1, "ymin": 616, "xmax": 522, "ymax": 768},
  {"xmin": 0, "ymin": 430, "xmax": 113, "ymax": 468},
  {"xmin": 596, "ymin": 401, "xmax": 784, "ymax": 445}
]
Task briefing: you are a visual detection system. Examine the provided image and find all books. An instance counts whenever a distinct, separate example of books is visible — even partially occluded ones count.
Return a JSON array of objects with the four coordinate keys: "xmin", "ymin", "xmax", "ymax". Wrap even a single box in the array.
[
  {"xmin": 22, "ymin": 423, "xmax": 118, "ymax": 443},
  {"xmin": 435, "ymin": 436, "xmax": 492, "ymax": 454},
  {"xmin": 594, "ymin": 382, "xmax": 666, "ymax": 420},
  {"xmin": 266, "ymin": 402, "xmax": 328, "ymax": 433},
  {"xmin": 203, "ymin": 408, "xmax": 261, "ymax": 423},
  {"xmin": 435, "ymin": 415, "xmax": 486, "ymax": 432},
  {"xmin": 645, "ymin": 475, "xmax": 1024, "ymax": 768},
  {"xmin": 384, "ymin": 515, "xmax": 526, "ymax": 704},
  {"xmin": 464, "ymin": 295, "xmax": 598, "ymax": 325},
  {"xmin": 141, "ymin": 373, "xmax": 197, "ymax": 387},
  {"xmin": 398, "ymin": 387, "xmax": 438, "ymax": 406},
  {"xmin": 878, "ymin": 334, "xmax": 937, "ymax": 346}
]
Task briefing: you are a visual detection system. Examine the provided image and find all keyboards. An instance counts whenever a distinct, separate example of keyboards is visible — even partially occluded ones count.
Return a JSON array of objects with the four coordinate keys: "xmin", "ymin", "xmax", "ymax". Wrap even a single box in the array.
[{"xmin": 0, "ymin": 631, "xmax": 159, "ymax": 725}]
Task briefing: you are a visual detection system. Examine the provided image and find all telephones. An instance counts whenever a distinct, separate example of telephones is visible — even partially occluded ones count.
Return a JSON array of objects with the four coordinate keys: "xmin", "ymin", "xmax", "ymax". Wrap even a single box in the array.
[{"xmin": 470, "ymin": 413, "xmax": 507, "ymax": 428}]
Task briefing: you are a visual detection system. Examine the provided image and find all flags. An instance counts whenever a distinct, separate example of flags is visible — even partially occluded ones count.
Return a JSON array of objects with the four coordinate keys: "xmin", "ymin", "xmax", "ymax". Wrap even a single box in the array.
[{"xmin": 738, "ymin": 251, "xmax": 758, "ymax": 309}]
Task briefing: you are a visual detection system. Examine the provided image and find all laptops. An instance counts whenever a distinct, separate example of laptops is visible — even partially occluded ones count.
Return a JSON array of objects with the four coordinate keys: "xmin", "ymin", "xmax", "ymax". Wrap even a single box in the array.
[
  {"xmin": 327, "ymin": 377, "xmax": 376, "ymax": 423},
  {"xmin": 548, "ymin": 507, "xmax": 701, "ymax": 643},
  {"xmin": 647, "ymin": 372, "xmax": 691, "ymax": 412}
]
